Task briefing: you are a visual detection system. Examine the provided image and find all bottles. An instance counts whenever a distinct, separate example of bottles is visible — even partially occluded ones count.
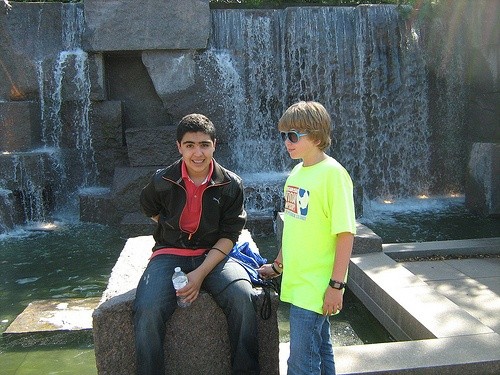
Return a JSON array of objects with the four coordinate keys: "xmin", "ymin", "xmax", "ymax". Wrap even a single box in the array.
[{"xmin": 171, "ymin": 267, "xmax": 191, "ymax": 307}]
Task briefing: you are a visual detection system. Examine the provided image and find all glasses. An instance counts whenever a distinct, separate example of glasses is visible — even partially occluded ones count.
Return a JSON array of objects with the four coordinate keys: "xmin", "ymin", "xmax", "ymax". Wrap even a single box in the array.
[{"xmin": 279, "ymin": 131, "xmax": 308, "ymax": 144}]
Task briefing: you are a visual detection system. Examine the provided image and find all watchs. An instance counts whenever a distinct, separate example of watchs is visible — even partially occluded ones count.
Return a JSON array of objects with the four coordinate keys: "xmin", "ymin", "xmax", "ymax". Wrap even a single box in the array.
[
  {"xmin": 329, "ymin": 279, "xmax": 347, "ymax": 290},
  {"xmin": 274, "ymin": 260, "xmax": 283, "ymax": 269}
]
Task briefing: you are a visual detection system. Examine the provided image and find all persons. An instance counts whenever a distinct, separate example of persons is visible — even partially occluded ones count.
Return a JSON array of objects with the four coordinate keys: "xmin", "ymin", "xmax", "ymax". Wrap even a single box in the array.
[
  {"xmin": 254, "ymin": 101, "xmax": 356, "ymax": 375},
  {"xmin": 132, "ymin": 112, "xmax": 260, "ymax": 375}
]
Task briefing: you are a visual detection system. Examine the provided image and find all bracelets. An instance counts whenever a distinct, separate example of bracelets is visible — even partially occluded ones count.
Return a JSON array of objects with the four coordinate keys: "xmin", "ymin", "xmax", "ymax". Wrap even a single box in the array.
[{"xmin": 271, "ymin": 263, "xmax": 281, "ymax": 275}]
